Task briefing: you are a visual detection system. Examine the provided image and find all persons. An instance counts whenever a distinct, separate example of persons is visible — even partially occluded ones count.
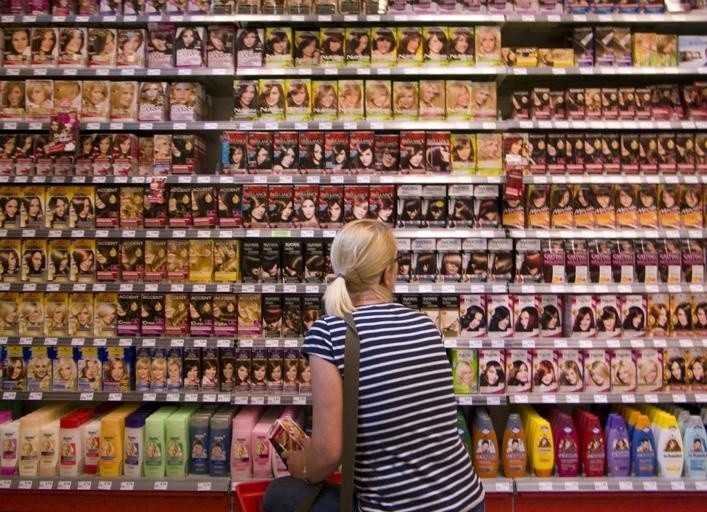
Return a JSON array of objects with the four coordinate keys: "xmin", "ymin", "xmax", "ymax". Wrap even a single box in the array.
[{"xmin": 278, "ymin": 219, "xmax": 486, "ymax": 512}]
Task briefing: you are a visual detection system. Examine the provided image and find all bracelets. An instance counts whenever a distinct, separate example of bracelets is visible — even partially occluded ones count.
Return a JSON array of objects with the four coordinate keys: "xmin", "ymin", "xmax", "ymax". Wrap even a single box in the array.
[{"xmin": 301, "ymin": 464, "xmax": 312, "ymax": 485}]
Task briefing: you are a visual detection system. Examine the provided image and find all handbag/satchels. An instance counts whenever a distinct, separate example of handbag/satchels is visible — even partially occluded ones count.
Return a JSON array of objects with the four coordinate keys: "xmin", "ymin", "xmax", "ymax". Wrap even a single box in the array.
[{"xmin": 262, "ymin": 476, "xmax": 357, "ymax": 511}]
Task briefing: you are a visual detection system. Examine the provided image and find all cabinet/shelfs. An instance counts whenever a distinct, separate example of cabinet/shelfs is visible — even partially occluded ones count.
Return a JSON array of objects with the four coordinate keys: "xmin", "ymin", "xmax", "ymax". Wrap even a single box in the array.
[{"xmin": 0, "ymin": 12, "xmax": 707, "ymax": 492}]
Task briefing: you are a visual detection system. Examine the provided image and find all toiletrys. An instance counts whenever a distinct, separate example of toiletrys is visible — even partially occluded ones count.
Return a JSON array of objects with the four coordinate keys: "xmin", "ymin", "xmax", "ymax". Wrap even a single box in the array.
[{"xmin": 0, "ymin": 407, "xmax": 707, "ymax": 477}]
